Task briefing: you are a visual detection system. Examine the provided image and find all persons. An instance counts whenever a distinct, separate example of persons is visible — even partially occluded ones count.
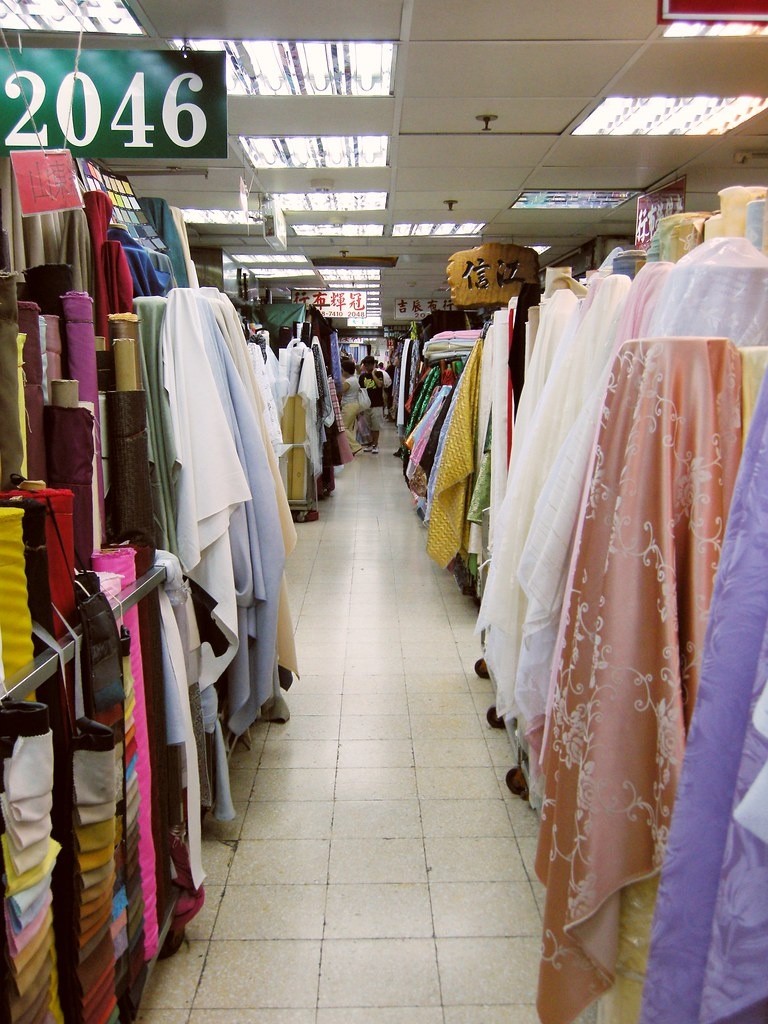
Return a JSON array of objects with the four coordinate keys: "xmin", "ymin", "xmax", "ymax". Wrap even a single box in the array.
[
  {"xmin": 340, "ymin": 361, "xmax": 362, "ymax": 456},
  {"xmin": 358, "ymin": 356, "xmax": 384, "ymax": 454},
  {"xmin": 360, "ymin": 359, "xmax": 385, "ymax": 419}
]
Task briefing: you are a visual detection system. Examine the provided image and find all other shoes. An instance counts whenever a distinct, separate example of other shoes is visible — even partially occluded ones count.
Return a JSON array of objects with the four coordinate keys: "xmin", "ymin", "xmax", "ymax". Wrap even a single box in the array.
[
  {"xmin": 363, "ymin": 446, "xmax": 374, "ymax": 452},
  {"xmin": 372, "ymin": 446, "xmax": 379, "ymax": 453},
  {"xmin": 352, "ymin": 446, "xmax": 362, "ymax": 456}
]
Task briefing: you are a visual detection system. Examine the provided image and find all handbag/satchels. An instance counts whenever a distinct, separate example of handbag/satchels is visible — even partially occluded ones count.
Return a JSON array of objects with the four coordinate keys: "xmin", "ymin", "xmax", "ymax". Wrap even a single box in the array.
[
  {"xmin": 357, "ymin": 383, "xmax": 371, "ymax": 414},
  {"xmin": 376, "ymin": 368, "xmax": 392, "ymax": 388}
]
